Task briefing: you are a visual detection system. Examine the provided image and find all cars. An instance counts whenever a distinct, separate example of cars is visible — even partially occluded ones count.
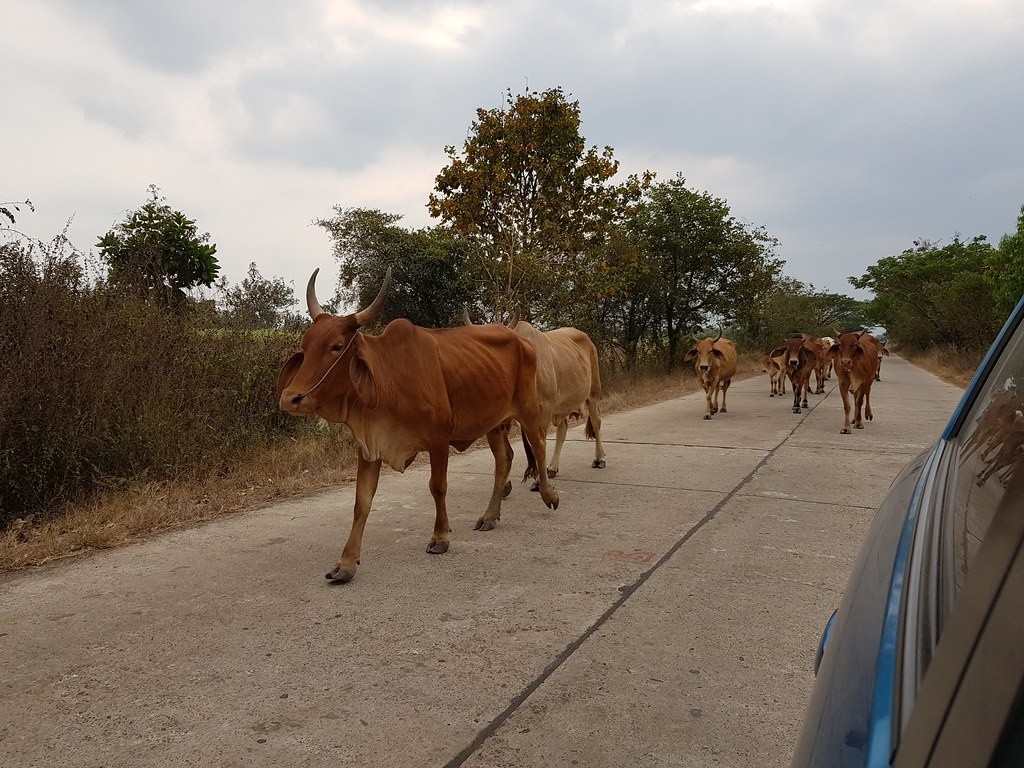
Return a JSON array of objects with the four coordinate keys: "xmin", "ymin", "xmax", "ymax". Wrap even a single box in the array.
[{"xmin": 787, "ymin": 286, "xmax": 1024, "ymax": 768}]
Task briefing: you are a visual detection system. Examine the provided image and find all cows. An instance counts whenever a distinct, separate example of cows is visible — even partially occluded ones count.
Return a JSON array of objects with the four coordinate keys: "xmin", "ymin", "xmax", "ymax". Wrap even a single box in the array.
[
  {"xmin": 762, "ymin": 328, "xmax": 890, "ymax": 434},
  {"xmin": 683, "ymin": 323, "xmax": 737, "ymax": 420},
  {"xmin": 276, "ymin": 266, "xmax": 606, "ymax": 585},
  {"xmin": 958, "ymin": 388, "xmax": 1024, "ymax": 490}
]
